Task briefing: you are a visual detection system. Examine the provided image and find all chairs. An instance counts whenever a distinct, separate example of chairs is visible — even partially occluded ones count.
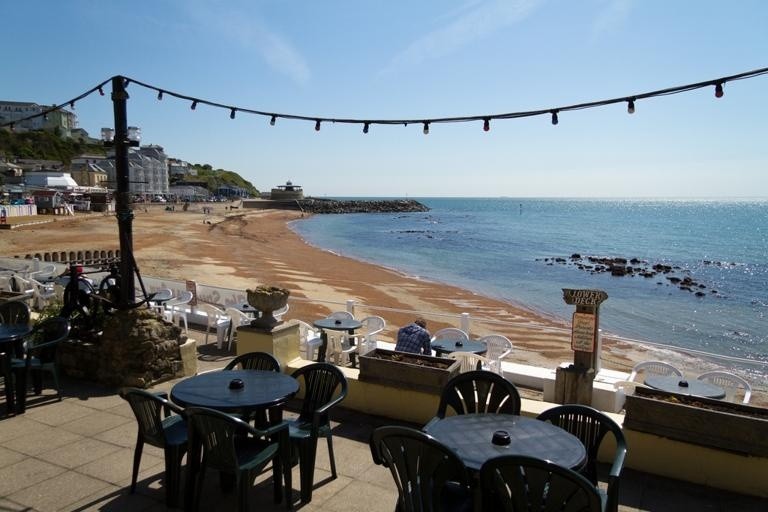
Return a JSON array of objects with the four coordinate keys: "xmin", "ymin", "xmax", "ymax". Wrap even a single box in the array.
[
  {"xmin": 281, "ymin": 362, "xmax": 350, "ymax": 504},
  {"xmin": 1, "ymin": 253, "xmax": 755, "ymax": 407},
  {"xmin": 117, "ymin": 386, "xmax": 183, "ymax": 503},
  {"xmin": 421, "ymin": 370, "xmax": 522, "ymax": 435},
  {"xmin": 470, "ymin": 453, "xmax": 604, "ymax": 511},
  {"xmin": 535, "ymin": 400, "xmax": 629, "ymax": 512},
  {"xmin": 367, "ymin": 424, "xmax": 470, "ymax": 512},
  {"xmin": 181, "ymin": 405, "xmax": 282, "ymax": 512},
  {"xmin": 223, "ymin": 352, "xmax": 280, "ymax": 374}
]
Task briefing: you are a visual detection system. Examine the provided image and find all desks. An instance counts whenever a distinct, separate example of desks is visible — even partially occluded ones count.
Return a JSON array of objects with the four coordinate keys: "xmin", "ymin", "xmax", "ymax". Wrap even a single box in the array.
[
  {"xmin": 422, "ymin": 412, "xmax": 588, "ymax": 470},
  {"xmin": 171, "ymin": 369, "xmax": 300, "ymax": 409}
]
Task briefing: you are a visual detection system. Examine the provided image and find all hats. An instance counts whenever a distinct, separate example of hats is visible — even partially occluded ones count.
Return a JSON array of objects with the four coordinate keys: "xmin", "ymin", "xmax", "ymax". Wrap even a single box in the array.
[{"xmin": 76, "ymin": 264, "xmax": 83, "ymax": 273}]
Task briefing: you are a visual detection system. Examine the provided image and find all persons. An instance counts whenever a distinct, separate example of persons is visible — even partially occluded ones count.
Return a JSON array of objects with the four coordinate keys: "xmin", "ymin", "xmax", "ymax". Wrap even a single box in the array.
[{"xmin": 396, "ymin": 316, "xmax": 431, "ymax": 356}]
